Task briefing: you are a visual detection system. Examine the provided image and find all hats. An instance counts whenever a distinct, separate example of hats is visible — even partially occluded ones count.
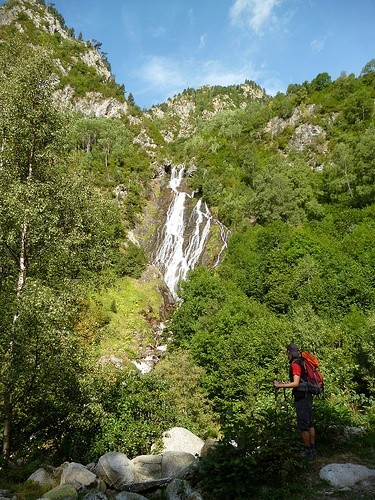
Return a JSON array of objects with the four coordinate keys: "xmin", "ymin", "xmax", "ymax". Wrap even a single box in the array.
[{"xmin": 285, "ymin": 344, "xmax": 299, "ymax": 357}]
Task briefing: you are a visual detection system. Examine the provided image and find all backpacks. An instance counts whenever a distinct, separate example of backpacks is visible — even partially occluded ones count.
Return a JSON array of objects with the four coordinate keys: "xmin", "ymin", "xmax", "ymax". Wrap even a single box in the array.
[{"xmin": 289, "ymin": 352, "xmax": 325, "ymax": 400}]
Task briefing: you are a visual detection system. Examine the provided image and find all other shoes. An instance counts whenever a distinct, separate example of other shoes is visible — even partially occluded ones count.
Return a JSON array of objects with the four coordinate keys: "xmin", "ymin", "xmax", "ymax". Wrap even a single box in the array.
[{"xmin": 294, "ymin": 448, "xmax": 316, "ymax": 460}]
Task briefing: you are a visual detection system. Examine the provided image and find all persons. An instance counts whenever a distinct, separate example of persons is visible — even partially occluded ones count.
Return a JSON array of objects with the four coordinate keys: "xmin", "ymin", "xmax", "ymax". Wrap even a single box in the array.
[{"xmin": 273, "ymin": 343, "xmax": 316, "ymax": 460}]
